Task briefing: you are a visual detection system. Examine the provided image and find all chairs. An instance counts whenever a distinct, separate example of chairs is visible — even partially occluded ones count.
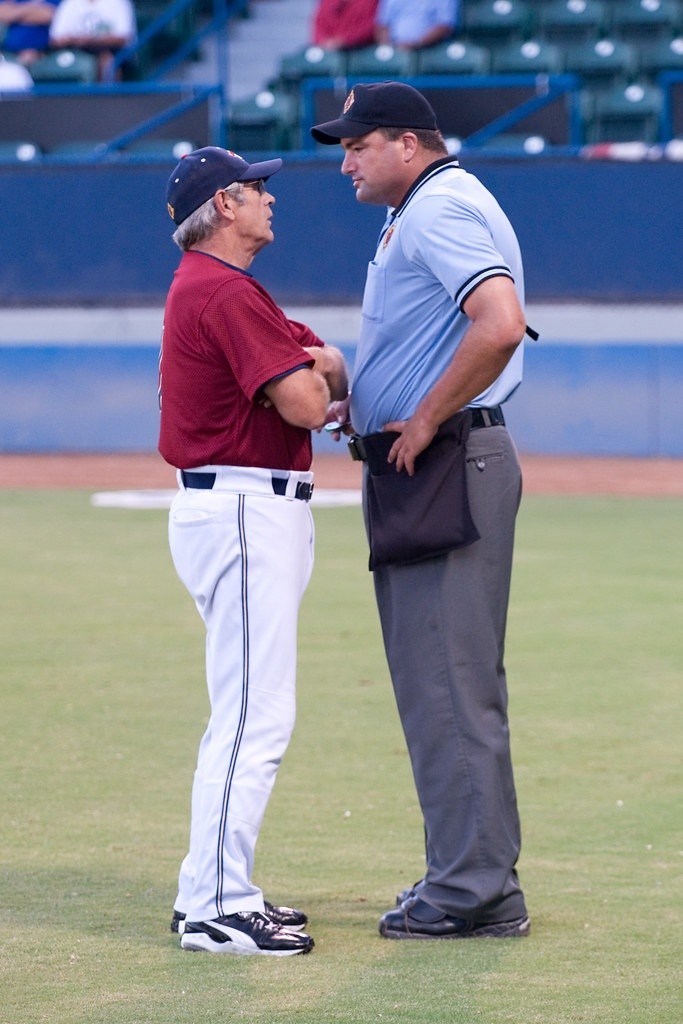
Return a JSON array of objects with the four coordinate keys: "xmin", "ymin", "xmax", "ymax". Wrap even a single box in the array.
[{"xmin": 0, "ymin": 1, "xmax": 682, "ymax": 160}]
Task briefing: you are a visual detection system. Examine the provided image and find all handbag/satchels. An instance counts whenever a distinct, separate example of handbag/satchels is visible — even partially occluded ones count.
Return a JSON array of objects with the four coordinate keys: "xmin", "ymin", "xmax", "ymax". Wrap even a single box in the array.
[{"xmin": 367, "ymin": 409, "xmax": 482, "ymax": 570}]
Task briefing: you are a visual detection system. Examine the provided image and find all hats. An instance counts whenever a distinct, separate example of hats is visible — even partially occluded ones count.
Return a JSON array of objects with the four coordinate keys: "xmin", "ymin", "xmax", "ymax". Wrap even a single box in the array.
[
  {"xmin": 311, "ymin": 79, "xmax": 438, "ymax": 145},
  {"xmin": 166, "ymin": 146, "xmax": 282, "ymax": 226}
]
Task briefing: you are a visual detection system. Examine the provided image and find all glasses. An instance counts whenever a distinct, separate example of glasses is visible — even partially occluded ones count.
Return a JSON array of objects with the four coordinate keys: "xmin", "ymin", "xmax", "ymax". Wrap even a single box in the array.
[{"xmin": 225, "ymin": 179, "xmax": 266, "ymax": 196}]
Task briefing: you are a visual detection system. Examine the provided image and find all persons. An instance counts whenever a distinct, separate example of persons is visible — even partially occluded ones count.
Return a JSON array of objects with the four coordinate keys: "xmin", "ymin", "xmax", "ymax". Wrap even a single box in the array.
[
  {"xmin": 157, "ymin": 146, "xmax": 349, "ymax": 958},
  {"xmin": 0, "ymin": 0, "xmax": 139, "ymax": 83},
  {"xmin": 310, "ymin": 80, "xmax": 532, "ymax": 941},
  {"xmin": 310, "ymin": 0, "xmax": 461, "ymax": 53}
]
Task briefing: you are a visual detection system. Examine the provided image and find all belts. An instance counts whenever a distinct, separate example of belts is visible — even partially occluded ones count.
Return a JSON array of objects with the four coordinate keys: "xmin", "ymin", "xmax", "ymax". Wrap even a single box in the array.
[
  {"xmin": 180, "ymin": 470, "xmax": 315, "ymax": 501},
  {"xmin": 346, "ymin": 403, "xmax": 506, "ymax": 460}
]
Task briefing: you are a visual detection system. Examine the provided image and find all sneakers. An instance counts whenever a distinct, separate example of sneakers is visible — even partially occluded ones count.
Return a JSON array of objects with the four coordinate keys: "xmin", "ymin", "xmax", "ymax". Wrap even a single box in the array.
[
  {"xmin": 379, "ymin": 896, "xmax": 531, "ymax": 939},
  {"xmin": 180, "ymin": 912, "xmax": 315, "ymax": 957},
  {"xmin": 171, "ymin": 901, "xmax": 307, "ymax": 932},
  {"xmin": 396, "ymin": 890, "xmax": 415, "ymax": 907}
]
